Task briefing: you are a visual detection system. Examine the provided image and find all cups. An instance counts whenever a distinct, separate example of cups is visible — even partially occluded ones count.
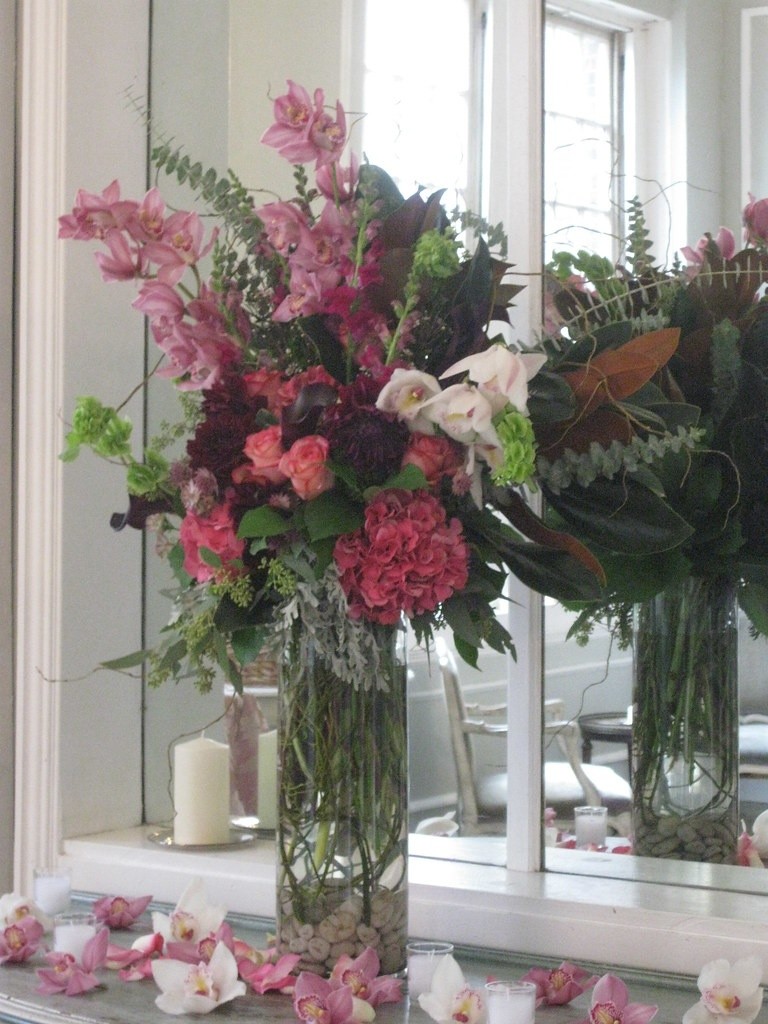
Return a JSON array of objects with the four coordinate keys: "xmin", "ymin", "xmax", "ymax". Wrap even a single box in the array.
[
  {"xmin": 405, "ymin": 941, "xmax": 453, "ymax": 1007},
  {"xmin": 484, "ymin": 981, "xmax": 537, "ymax": 1024},
  {"xmin": 573, "ymin": 805, "xmax": 609, "ymax": 852},
  {"xmin": 32, "ymin": 866, "xmax": 73, "ymax": 919},
  {"xmin": 54, "ymin": 911, "xmax": 96, "ymax": 967}
]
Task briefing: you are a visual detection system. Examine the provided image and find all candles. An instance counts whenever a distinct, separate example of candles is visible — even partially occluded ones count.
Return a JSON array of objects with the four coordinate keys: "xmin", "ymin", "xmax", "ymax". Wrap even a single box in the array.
[
  {"xmin": 171, "ymin": 725, "xmax": 235, "ymax": 848},
  {"xmin": 487, "ymin": 986, "xmax": 534, "ymax": 1023},
  {"xmin": 406, "ymin": 948, "xmax": 445, "ymax": 1000},
  {"xmin": 255, "ymin": 727, "xmax": 285, "ymax": 835}
]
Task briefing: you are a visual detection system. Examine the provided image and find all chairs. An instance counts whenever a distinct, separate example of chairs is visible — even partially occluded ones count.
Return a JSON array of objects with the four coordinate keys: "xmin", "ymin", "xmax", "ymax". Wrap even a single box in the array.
[{"xmin": 433, "ymin": 642, "xmax": 641, "ymax": 845}]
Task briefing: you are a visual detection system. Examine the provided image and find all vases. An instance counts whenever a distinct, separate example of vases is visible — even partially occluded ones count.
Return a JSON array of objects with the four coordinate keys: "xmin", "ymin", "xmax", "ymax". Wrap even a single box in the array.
[
  {"xmin": 618, "ymin": 578, "xmax": 740, "ymax": 873},
  {"xmin": 273, "ymin": 600, "xmax": 407, "ymax": 983}
]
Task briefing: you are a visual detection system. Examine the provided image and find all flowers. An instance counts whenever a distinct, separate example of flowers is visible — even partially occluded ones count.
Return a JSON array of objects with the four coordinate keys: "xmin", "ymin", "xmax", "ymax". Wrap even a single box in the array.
[
  {"xmin": 493, "ymin": 184, "xmax": 764, "ymax": 619},
  {"xmin": 57, "ymin": 72, "xmax": 524, "ymax": 934}
]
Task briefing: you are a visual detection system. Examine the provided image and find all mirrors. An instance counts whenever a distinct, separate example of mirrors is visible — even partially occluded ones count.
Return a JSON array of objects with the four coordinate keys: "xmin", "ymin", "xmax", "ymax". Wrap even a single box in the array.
[{"xmin": 47, "ymin": 0, "xmax": 768, "ymax": 896}]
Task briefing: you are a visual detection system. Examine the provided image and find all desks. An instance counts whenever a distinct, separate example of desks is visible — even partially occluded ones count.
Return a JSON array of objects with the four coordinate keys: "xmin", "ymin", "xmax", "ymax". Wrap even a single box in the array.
[{"xmin": 576, "ymin": 710, "xmax": 670, "ymax": 793}]
